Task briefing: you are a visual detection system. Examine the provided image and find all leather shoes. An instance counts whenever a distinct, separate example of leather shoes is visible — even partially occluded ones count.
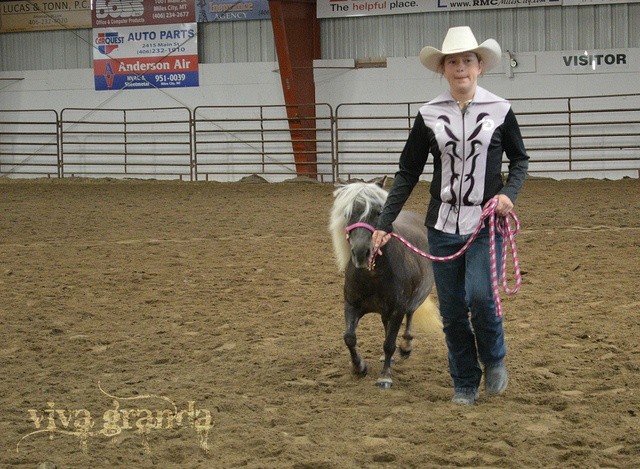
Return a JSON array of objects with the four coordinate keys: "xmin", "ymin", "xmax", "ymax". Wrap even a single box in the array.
[
  {"xmin": 485, "ymin": 363, "xmax": 508, "ymax": 396},
  {"xmin": 451, "ymin": 390, "xmax": 480, "ymax": 408}
]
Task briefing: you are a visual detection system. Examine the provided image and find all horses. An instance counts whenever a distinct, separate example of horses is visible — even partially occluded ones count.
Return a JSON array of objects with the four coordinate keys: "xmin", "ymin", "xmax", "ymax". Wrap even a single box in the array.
[{"xmin": 326, "ymin": 174, "xmax": 443, "ymax": 391}]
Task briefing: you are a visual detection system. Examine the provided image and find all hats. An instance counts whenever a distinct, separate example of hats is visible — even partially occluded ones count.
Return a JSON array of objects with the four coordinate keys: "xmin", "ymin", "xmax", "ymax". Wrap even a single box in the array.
[{"xmin": 419, "ymin": 27, "xmax": 501, "ymax": 74}]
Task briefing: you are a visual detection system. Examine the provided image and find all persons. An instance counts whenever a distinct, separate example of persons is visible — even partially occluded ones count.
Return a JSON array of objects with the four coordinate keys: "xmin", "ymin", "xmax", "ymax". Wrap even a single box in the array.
[{"xmin": 373, "ymin": 27, "xmax": 530, "ymax": 406}]
